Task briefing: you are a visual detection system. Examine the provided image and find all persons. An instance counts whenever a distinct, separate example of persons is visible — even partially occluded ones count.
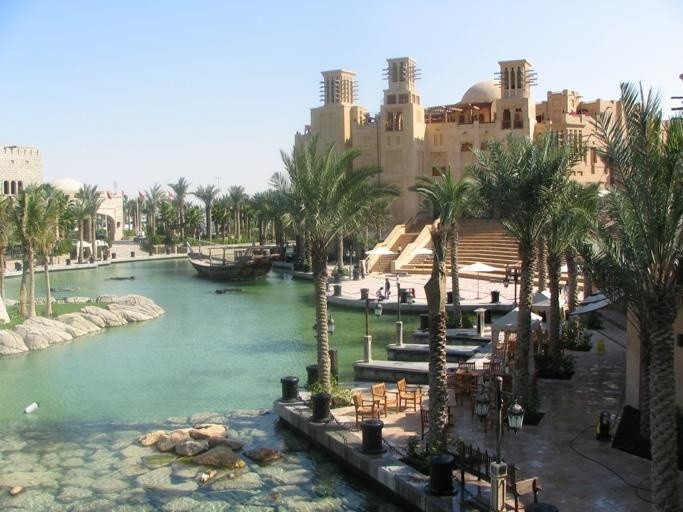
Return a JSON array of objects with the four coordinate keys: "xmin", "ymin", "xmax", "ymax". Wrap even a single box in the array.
[
  {"xmin": 353, "ymin": 264, "xmax": 365, "ymax": 280},
  {"xmin": 411, "ymin": 288, "xmax": 416, "ymax": 302},
  {"xmin": 376, "ymin": 278, "xmax": 390, "ymax": 300},
  {"xmin": 397, "ymin": 243, "xmax": 402, "ymax": 256}
]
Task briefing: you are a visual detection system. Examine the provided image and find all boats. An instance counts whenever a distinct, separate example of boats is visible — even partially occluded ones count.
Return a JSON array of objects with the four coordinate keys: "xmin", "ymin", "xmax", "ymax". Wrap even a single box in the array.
[{"xmin": 186, "ymin": 241, "xmax": 280, "ymax": 280}]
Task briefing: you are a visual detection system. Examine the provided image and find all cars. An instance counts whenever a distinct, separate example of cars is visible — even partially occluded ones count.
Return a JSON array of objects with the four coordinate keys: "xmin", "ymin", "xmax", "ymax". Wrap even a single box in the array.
[{"xmin": 284, "ymin": 245, "xmax": 297, "ymax": 261}]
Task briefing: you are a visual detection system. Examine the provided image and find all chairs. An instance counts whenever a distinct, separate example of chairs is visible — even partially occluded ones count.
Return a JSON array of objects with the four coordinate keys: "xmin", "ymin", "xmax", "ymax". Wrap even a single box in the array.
[
  {"xmin": 421, "ymin": 408, "xmax": 430, "ymax": 439},
  {"xmin": 446, "ymin": 362, "xmax": 501, "ymax": 423},
  {"xmin": 485, "ymin": 400, "xmax": 512, "ymax": 436},
  {"xmin": 353, "ymin": 391, "xmax": 380, "ymax": 427},
  {"xmin": 397, "ymin": 377, "xmax": 423, "ymax": 412},
  {"xmin": 371, "ymin": 383, "xmax": 399, "ymax": 417}
]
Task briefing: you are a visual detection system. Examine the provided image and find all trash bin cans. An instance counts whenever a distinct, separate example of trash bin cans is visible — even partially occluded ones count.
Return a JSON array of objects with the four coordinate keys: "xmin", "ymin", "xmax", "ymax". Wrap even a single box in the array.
[
  {"xmin": 596, "ymin": 411, "xmax": 611, "ymax": 441},
  {"xmin": 399, "ymin": 289, "xmax": 407, "ymax": 303}
]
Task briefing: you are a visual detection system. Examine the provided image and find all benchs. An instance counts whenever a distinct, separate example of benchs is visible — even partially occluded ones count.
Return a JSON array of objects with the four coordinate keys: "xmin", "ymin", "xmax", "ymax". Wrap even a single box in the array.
[{"xmin": 457, "ymin": 443, "xmax": 542, "ymax": 512}]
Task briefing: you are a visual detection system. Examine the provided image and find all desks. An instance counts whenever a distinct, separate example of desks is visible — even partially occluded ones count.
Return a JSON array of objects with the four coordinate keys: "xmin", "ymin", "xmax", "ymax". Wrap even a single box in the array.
[{"xmin": 446, "ymin": 389, "xmax": 456, "ymax": 426}]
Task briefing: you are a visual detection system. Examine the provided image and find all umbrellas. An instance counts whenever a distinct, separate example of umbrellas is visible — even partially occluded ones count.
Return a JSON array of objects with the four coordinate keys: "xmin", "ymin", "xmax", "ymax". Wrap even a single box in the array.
[
  {"xmin": 364, "ymin": 247, "xmax": 394, "ymax": 272},
  {"xmin": 407, "ymin": 248, "xmax": 433, "ymax": 274},
  {"xmin": 458, "ymin": 262, "xmax": 501, "ymax": 298},
  {"xmin": 557, "ymin": 263, "xmax": 600, "ymax": 279}
]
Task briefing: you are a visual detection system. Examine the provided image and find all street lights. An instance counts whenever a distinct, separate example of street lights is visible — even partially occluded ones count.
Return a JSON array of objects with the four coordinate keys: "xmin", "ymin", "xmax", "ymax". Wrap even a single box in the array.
[
  {"xmin": 313, "ymin": 314, "xmax": 335, "ymax": 340},
  {"xmin": 366, "ymin": 281, "xmax": 413, "ymax": 335},
  {"xmin": 503, "ymin": 268, "xmax": 518, "ymax": 302},
  {"xmin": 495, "ymin": 376, "xmax": 524, "ymax": 464},
  {"xmin": 346, "ymin": 248, "xmax": 356, "ymax": 278}
]
